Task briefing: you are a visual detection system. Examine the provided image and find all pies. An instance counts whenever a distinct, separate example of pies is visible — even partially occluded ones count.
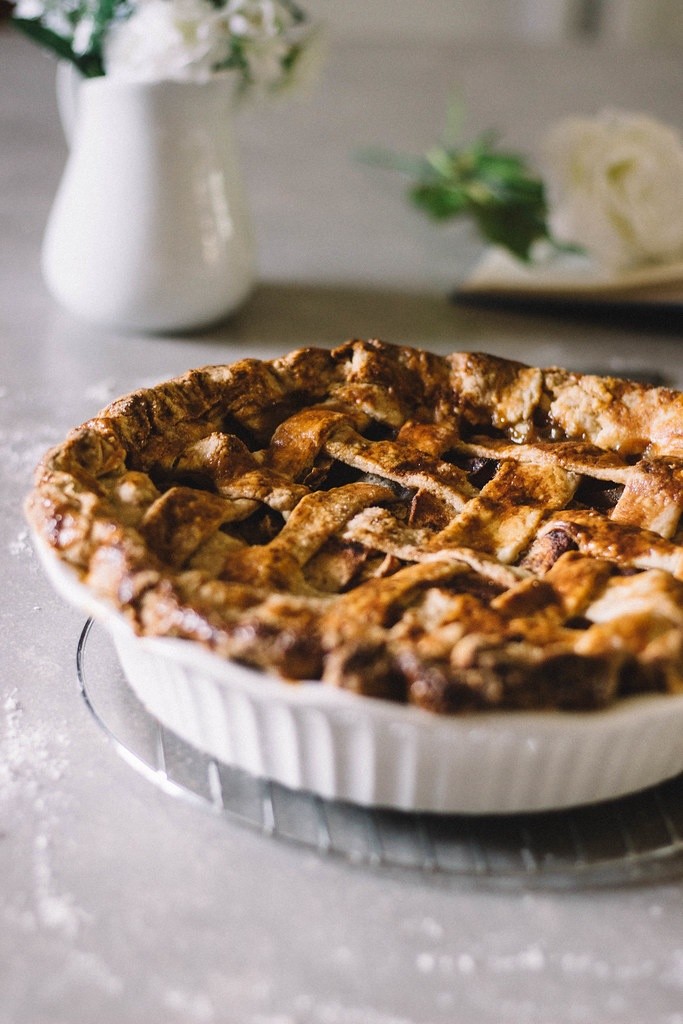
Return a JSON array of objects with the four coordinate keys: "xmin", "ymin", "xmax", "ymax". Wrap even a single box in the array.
[{"xmin": 31, "ymin": 340, "xmax": 683, "ymax": 715}]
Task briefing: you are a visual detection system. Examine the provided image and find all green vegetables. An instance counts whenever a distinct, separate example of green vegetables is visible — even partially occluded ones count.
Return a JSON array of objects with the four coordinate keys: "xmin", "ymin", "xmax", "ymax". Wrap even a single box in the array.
[{"xmin": 413, "ymin": 125, "xmax": 548, "ymax": 264}]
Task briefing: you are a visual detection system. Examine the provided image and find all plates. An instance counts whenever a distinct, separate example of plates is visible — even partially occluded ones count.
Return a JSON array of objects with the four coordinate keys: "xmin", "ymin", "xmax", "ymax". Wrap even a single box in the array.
[{"xmin": 75, "ymin": 615, "xmax": 683, "ymax": 879}]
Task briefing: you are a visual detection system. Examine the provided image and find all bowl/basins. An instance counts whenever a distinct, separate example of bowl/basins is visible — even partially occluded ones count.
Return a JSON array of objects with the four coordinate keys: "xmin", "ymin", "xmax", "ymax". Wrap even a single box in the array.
[{"xmin": 22, "ymin": 505, "xmax": 683, "ymax": 814}]
